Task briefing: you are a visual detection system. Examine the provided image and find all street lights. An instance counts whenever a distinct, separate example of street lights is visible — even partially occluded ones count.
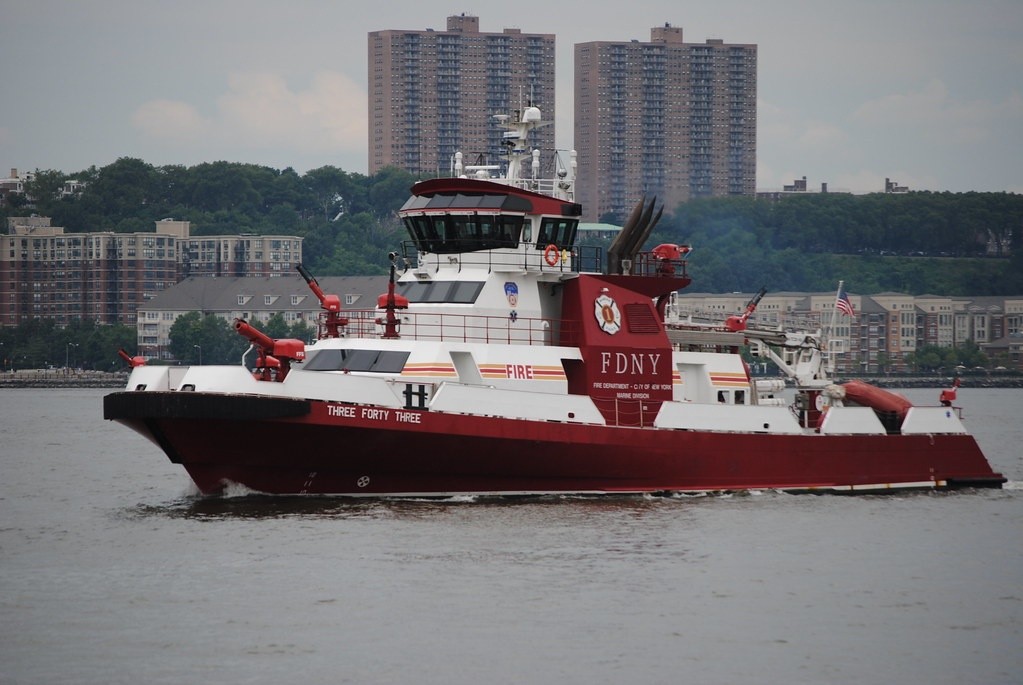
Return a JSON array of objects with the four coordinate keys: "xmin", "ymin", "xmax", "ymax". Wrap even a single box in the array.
[{"xmin": 193, "ymin": 344, "xmax": 202, "ymax": 366}]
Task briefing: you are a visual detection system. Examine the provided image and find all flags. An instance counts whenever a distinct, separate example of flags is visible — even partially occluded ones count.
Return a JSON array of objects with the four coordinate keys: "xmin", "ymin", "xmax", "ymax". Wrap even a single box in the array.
[{"xmin": 836, "ymin": 285, "xmax": 856, "ymax": 319}]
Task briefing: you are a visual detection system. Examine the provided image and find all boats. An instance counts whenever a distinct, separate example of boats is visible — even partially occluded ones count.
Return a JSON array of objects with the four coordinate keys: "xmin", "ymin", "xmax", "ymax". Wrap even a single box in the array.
[{"xmin": 100, "ymin": 78, "xmax": 1010, "ymax": 503}]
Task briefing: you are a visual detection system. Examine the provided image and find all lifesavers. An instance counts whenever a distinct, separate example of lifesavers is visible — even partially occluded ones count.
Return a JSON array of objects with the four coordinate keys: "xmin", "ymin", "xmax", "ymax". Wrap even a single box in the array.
[{"xmin": 545, "ymin": 244, "xmax": 560, "ymax": 266}]
